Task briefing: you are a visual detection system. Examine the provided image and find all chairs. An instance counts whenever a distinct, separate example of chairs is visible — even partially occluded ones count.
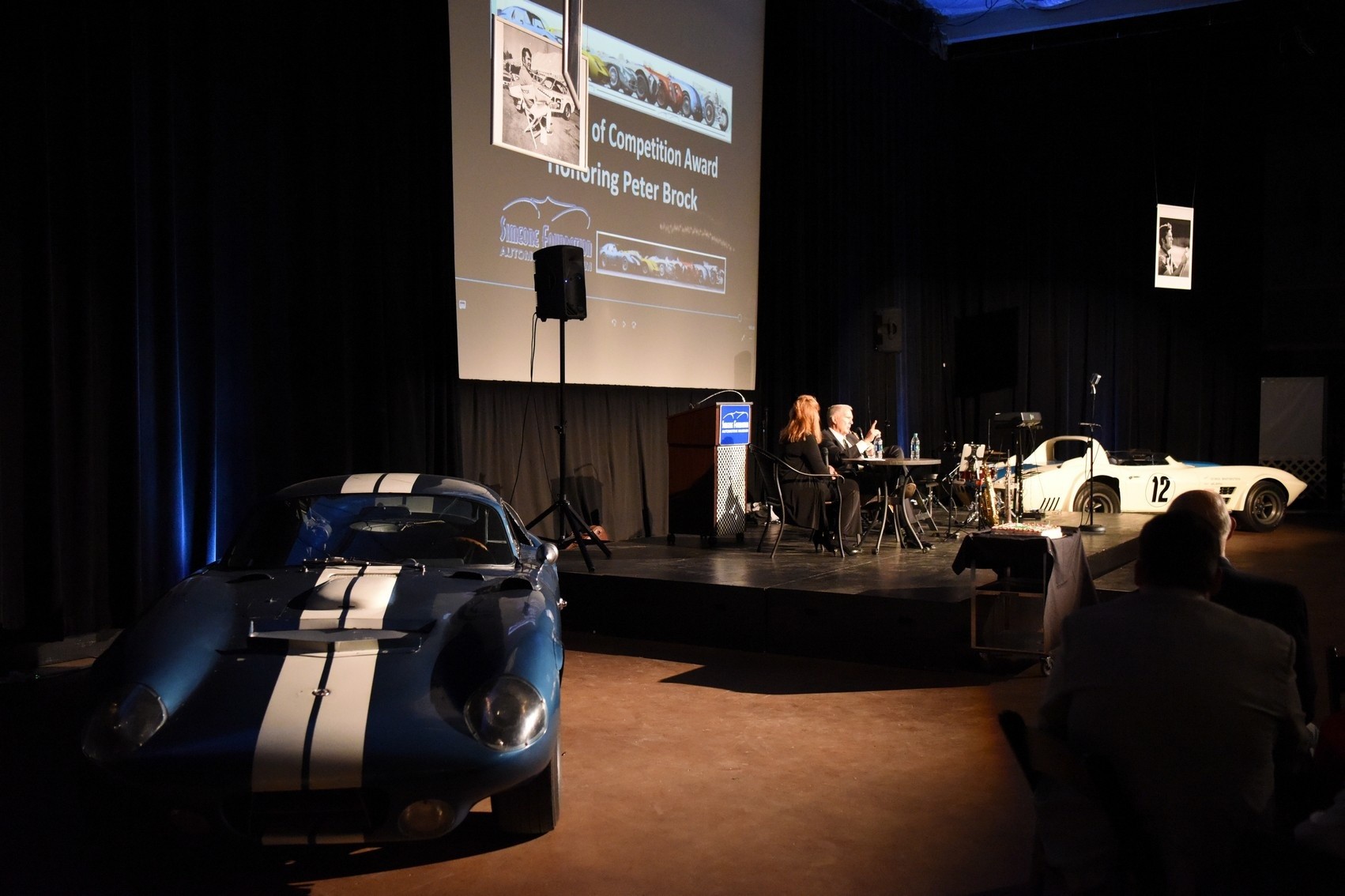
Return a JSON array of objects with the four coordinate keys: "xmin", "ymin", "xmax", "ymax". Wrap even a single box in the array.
[
  {"xmin": 748, "ymin": 444, "xmax": 845, "ymax": 559},
  {"xmin": 394, "ymin": 519, "xmax": 463, "ymax": 558}
]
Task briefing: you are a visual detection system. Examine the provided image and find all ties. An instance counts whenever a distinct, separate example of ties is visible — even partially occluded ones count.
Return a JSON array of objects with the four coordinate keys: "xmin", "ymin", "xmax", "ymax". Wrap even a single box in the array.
[{"xmin": 843, "ymin": 439, "xmax": 849, "ymax": 449}]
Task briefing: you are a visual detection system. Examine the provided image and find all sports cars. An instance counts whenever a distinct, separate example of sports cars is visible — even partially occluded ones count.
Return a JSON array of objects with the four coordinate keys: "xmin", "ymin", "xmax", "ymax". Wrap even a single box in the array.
[
  {"xmin": 64, "ymin": 471, "xmax": 564, "ymax": 850},
  {"xmin": 985, "ymin": 435, "xmax": 1309, "ymax": 533}
]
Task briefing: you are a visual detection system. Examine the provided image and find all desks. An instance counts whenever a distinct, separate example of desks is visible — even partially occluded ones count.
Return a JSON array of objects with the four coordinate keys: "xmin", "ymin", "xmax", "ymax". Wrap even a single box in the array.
[
  {"xmin": 841, "ymin": 456, "xmax": 941, "ymax": 556},
  {"xmin": 952, "ymin": 534, "xmax": 1096, "ymax": 656}
]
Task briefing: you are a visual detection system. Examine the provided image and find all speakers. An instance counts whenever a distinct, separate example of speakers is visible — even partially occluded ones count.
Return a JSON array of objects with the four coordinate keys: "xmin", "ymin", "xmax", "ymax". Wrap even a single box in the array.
[{"xmin": 531, "ymin": 244, "xmax": 588, "ymax": 322}]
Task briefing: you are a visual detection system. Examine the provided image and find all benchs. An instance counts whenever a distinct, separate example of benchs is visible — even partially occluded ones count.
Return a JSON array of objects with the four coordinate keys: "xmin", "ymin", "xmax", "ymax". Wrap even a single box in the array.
[{"xmin": 337, "ymin": 504, "xmax": 481, "ymax": 542}]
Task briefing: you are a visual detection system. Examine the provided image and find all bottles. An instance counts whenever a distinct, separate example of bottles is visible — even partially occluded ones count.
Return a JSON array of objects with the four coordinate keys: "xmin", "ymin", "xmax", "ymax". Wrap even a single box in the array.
[
  {"xmin": 910, "ymin": 433, "xmax": 920, "ymax": 461},
  {"xmin": 874, "ymin": 435, "xmax": 883, "ymax": 459}
]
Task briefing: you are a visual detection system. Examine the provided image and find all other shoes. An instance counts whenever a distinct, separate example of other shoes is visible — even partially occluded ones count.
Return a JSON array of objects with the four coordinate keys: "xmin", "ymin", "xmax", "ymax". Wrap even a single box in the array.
[
  {"xmin": 906, "ymin": 537, "xmax": 934, "ymax": 548},
  {"xmin": 891, "ymin": 483, "xmax": 916, "ymax": 501}
]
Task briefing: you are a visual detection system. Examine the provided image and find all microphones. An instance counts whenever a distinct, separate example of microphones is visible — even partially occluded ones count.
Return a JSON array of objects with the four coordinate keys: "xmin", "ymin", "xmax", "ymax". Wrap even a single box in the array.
[
  {"xmin": 695, "ymin": 390, "xmax": 745, "ymax": 407},
  {"xmin": 1090, "ymin": 373, "xmax": 1101, "ymax": 385},
  {"xmin": 856, "ymin": 427, "xmax": 869, "ymax": 458},
  {"xmin": 821, "ymin": 447, "xmax": 829, "ymax": 467},
  {"xmin": 1078, "ymin": 422, "xmax": 1100, "ymax": 428}
]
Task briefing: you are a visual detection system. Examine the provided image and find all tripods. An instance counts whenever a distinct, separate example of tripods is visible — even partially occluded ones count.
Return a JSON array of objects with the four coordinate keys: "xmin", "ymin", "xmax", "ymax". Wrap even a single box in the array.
[
  {"xmin": 526, "ymin": 320, "xmax": 613, "ymax": 574},
  {"xmin": 946, "ymin": 468, "xmax": 995, "ymax": 540}
]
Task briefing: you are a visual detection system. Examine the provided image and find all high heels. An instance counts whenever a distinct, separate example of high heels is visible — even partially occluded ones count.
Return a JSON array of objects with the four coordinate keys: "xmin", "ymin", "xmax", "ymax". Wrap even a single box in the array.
[
  {"xmin": 824, "ymin": 533, "xmax": 861, "ymax": 556},
  {"xmin": 813, "ymin": 530, "xmax": 837, "ymax": 554}
]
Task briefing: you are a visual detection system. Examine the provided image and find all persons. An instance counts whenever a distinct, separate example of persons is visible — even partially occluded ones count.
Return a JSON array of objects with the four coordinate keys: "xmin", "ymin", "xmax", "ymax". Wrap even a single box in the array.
[
  {"xmin": 1034, "ymin": 512, "xmax": 1317, "ymax": 895},
  {"xmin": 1166, "ymin": 490, "xmax": 1316, "ymax": 727},
  {"xmin": 777, "ymin": 395, "xmax": 862, "ymax": 556},
  {"xmin": 1157, "ymin": 223, "xmax": 1190, "ymax": 276},
  {"xmin": 519, "ymin": 48, "xmax": 553, "ymax": 134},
  {"xmin": 818, "ymin": 403, "xmax": 936, "ymax": 549}
]
